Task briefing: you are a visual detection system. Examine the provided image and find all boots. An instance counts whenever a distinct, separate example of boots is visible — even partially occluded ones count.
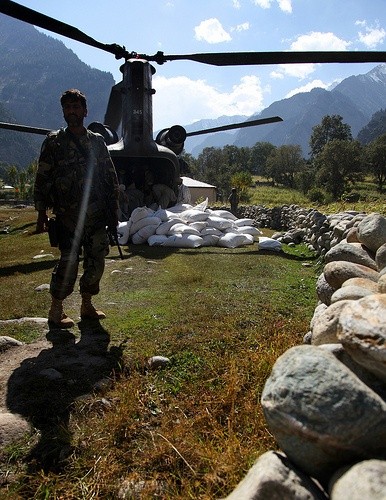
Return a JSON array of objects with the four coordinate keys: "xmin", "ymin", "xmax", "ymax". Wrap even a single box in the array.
[
  {"xmin": 47, "ymin": 296, "xmax": 77, "ymax": 328},
  {"xmin": 79, "ymin": 292, "xmax": 106, "ymax": 319}
]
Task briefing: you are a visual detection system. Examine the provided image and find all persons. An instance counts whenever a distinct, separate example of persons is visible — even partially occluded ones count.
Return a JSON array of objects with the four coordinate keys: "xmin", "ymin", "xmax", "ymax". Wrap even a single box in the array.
[
  {"xmin": 34, "ymin": 89, "xmax": 120, "ymax": 328},
  {"xmin": 124, "ymin": 177, "xmax": 144, "ymax": 217},
  {"xmin": 145, "ymin": 183, "xmax": 178, "ymax": 208},
  {"xmin": 176, "ymin": 177, "xmax": 191, "ymax": 204},
  {"xmin": 229, "ymin": 188, "xmax": 240, "ymax": 215}
]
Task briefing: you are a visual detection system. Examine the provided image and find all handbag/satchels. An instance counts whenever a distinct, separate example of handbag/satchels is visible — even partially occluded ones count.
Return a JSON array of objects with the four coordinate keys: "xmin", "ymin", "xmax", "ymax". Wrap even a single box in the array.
[
  {"xmin": 45, "ymin": 216, "xmax": 60, "ymax": 246},
  {"xmin": 98, "ymin": 174, "xmax": 119, "ymax": 233}
]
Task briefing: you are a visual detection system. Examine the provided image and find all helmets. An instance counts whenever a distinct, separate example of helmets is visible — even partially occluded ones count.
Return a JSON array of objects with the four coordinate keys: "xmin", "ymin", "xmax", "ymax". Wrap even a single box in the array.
[{"xmin": 232, "ymin": 188, "xmax": 237, "ymax": 192}]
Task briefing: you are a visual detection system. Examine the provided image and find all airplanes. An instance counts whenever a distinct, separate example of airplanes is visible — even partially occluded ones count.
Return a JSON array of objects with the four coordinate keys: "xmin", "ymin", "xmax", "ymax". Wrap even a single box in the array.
[{"xmin": 0, "ymin": 0, "xmax": 386, "ymax": 223}]
